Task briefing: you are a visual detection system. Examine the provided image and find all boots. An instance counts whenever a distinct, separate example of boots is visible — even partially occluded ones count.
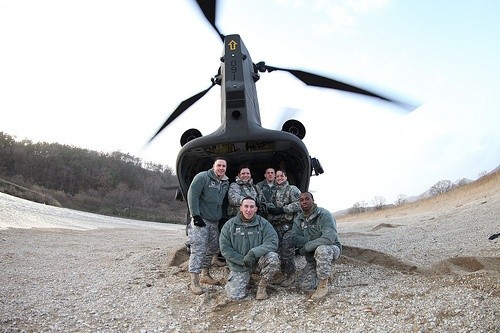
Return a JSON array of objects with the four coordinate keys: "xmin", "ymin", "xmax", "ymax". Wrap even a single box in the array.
[
  {"xmin": 199, "ymin": 268, "xmax": 220, "ymax": 284},
  {"xmin": 280, "ymin": 272, "xmax": 295, "ymax": 287},
  {"xmin": 211, "ymin": 255, "xmax": 226, "ymax": 266},
  {"xmin": 190, "ymin": 273, "xmax": 202, "ymax": 294},
  {"xmin": 311, "ymin": 279, "xmax": 328, "ymax": 299},
  {"xmin": 271, "ymin": 273, "xmax": 286, "ymax": 285},
  {"xmin": 256, "ymin": 278, "xmax": 267, "ymax": 299}
]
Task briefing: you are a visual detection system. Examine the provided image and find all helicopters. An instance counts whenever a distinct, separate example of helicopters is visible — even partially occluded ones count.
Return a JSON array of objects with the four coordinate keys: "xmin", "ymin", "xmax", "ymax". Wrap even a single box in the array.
[{"xmin": 147, "ymin": 0, "xmax": 418, "ymax": 266}]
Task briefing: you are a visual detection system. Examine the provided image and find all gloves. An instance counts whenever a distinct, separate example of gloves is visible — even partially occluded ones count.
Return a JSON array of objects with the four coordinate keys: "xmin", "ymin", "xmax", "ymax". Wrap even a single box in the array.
[
  {"xmin": 299, "ymin": 247, "xmax": 307, "ymax": 256},
  {"xmin": 242, "ymin": 252, "xmax": 257, "ymax": 268},
  {"xmin": 268, "ymin": 207, "xmax": 284, "ymax": 215},
  {"xmin": 193, "ymin": 215, "xmax": 206, "ymax": 227},
  {"xmin": 266, "ymin": 203, "xmax": 275, "ymax": 208},
  {"xmin": 310, "ymin": 230, "xmax": 322, "ymax": 240}
]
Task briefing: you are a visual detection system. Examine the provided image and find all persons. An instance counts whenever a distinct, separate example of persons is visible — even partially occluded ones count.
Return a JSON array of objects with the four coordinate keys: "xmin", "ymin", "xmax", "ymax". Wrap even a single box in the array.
[
  {"xmin": 219, "ymin": 196, "xmax": 280, "ymax": 300},
  {"xmin": 255, "ymin": 167, "xmax": 276, "ymax": 198},
  {"xmin": 270, "ymin": 170, "xmax": 303, "ymax": 287},
  {"xmin": 291, "ymin": 192, "xmax": 342, "ymax": 299},
  {"xmin": 187, "ymin": 157, "xmax": 231, "ymax": 295},
  {"xmin": 228, "ymin": 167, "xmax": 257, "ymax": 220}
]
